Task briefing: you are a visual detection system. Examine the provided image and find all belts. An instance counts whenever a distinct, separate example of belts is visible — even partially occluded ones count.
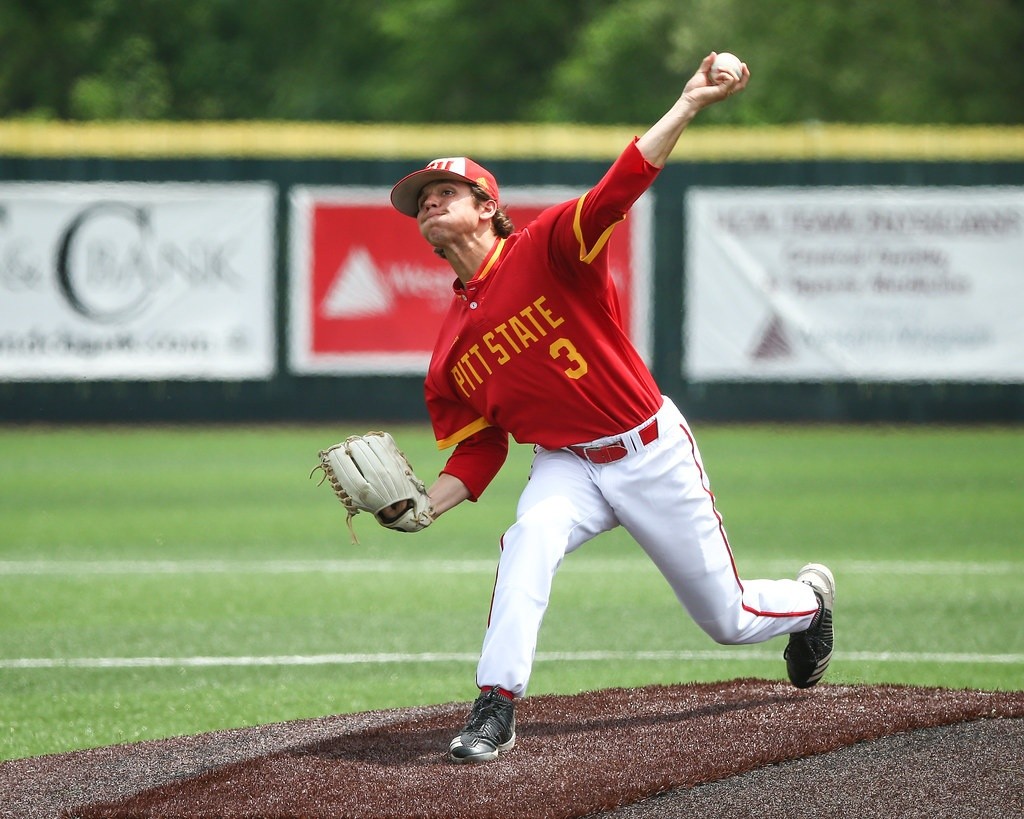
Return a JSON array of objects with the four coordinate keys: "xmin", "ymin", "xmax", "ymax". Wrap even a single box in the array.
[{"xmin": 565, "ymin": 418, "xmax": 658, "ymax": 465}]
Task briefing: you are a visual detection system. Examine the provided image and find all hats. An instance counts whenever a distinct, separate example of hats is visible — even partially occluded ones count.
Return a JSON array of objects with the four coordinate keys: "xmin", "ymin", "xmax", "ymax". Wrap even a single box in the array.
[{"xmin": 389, "ymin": 157, "xmax": 499, "ymax": 218}]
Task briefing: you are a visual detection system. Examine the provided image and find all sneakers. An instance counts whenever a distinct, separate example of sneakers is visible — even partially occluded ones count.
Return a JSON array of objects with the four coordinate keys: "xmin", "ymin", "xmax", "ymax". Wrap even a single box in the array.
[
  {"xmin": 448, "ymin": 685, "xmax": 516, "ymax": 763},
  {"xmin": 783, "ymin": 564, "xmax": 834, "ymax": 689}
]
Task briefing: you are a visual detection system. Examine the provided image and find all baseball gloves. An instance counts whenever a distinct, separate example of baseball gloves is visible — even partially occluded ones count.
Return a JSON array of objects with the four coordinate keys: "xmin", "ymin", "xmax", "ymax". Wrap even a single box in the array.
[{"xmin": 317, "ymin": 431, "xmax": 432, "ymax": 534}]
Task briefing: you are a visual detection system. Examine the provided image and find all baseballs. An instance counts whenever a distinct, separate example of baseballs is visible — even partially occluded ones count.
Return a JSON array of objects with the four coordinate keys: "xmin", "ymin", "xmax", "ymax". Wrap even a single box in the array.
[{"xmin": 711, "ymin": 49, "xmax": 743, "ymax": 88}]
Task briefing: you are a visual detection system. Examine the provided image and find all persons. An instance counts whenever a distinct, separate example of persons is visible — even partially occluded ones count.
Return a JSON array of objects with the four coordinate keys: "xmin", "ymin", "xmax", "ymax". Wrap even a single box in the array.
[{"xmin": 320, "ymin": 51, "xmax": 833, "ymax": 762}]
